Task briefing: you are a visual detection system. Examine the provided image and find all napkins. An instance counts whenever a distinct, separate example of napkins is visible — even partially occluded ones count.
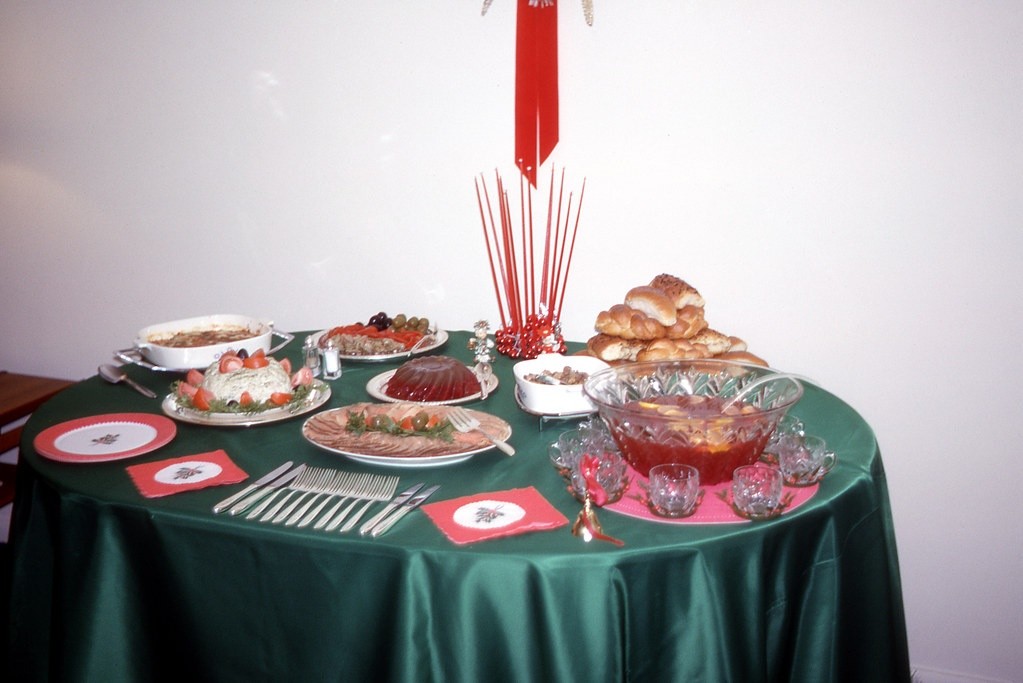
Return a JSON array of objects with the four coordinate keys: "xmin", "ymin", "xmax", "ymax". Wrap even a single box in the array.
[
  {"xmin": 124, "ymin": 451, "xmax": 250, "ymax": 499},
  {"xmin": 420, "ymin": 485, "xmax": 570, "ymax": 545}
]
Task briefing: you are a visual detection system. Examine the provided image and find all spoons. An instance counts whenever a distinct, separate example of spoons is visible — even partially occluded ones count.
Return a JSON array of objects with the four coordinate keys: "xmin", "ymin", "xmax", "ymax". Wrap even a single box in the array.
[{"xmin": 98, "ymin": 365, "xmax": 157, "ymax": 398}]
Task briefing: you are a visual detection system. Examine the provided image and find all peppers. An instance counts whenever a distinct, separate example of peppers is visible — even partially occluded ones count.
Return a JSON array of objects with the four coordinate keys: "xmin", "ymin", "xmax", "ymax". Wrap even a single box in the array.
[{"xmin": 328, "ymin": 323, "xmax": 429, "ymax": 348}]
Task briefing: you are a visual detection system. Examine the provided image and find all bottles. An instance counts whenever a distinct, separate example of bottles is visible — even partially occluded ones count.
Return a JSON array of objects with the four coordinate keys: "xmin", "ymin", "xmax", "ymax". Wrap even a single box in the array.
[
  {"xmin": 302, "ymin": 335, "xmax": 320, "ymax": 378},
  {"xmin": 322, "ymin": 339, "xmax": 342, "ymax": 380}
]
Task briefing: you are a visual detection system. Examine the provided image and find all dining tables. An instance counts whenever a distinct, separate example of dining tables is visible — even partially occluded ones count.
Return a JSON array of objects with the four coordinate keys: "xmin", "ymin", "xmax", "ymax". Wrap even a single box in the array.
[{"xmin": 0, "ymin": 329, "xmax": 914, "ymax": 683}]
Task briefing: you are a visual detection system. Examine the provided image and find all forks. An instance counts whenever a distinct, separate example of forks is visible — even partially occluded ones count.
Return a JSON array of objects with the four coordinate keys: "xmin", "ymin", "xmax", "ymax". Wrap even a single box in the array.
[
  {"xmin": 244, "ymin": 467, "xmax": 399, "ymax": 533},
  {"xmin": 446, "ymin": 407, "xmax": 515, "ymax": 456}
]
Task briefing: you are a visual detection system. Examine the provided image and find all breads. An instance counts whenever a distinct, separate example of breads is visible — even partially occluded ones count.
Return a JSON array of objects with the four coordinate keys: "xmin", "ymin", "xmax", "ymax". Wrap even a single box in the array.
[{"xmin": 571, "ymin": 274, "xmax": 769, "ymax": 374}]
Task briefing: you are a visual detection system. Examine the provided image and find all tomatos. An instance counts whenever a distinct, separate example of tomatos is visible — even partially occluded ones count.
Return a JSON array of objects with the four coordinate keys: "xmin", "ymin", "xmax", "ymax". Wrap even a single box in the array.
[{"xmin": 364, "ymin": 412, "xmax": 440, "ymax": 431}]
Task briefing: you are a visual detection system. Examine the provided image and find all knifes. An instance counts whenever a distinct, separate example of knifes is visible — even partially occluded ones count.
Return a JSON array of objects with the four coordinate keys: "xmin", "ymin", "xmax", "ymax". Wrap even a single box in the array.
[
  {"xmin": 359, "ymin": 483, "xmax": 441, "ymax": 536},
  {"xmin": 213, "ymin": 461, "xmax": 308, "ymax": 516}
]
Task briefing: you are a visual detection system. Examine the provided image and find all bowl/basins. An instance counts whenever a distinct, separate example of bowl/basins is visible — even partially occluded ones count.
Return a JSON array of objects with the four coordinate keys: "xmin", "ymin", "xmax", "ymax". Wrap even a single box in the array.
[
  {"xmin": 513, "ymin": 354, "xmax": 616, "ymax": 414},
  {"xmin": 134, "ymin": 314, "xmax": 272, "ymax": 369},
  {"xmin": 582, "ymin": 356, "xmax": 803, "ymax": 484}
]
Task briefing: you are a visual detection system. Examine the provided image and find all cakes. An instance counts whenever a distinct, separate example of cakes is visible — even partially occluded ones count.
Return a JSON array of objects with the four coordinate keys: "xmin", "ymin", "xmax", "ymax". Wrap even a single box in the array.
[{"xmin": 170, "ymin": 347, "xmax": 317, "ymax": 415}]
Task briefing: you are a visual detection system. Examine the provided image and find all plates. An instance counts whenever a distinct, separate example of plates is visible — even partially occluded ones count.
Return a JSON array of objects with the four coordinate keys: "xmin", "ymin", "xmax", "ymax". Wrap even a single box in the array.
[
  {"xmin": 302, "ymin": 406, "xmax": 512, "ymax": 468},
  {"xmin": 310, "ymin": 329, "xmax": 449, "ymax": 362},
  {"xmin": 33, "ymin": 413, "xmax": 176, "ymax": 461},
  {"xmin": 366, "ymin": 366, "xmax": 499, "ymax": 405},
  {"xmin": 162, "ymin": 378, "xmax": 332, "ymax": 427}
]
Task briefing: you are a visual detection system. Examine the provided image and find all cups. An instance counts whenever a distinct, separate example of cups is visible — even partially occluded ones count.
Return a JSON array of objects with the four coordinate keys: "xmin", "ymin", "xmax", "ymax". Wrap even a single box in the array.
[
  {"xmin": 732, "ymin": 466, "xmax": 782, "ymax": 514},
  {"xmin": 647, "ymin": 463, "xmax": 699, "ymax": 516},
  {"xmin": 547, "ymin": 428, "xmax": 605, "ymax": 471},
  {"xmin": 780, "ymin": 435, "xmax": 836, "ymax": 486},
  {"xmin": 578, "ymin": 413, "xmax": 619, "ymax": 452},
  {"xmin": 571, "ymin": 450, "xmax": 623, "ymax": 505},
  {"xmin": 762, "ymin": 414, "xmax": 804, "ymax": 463}
]
